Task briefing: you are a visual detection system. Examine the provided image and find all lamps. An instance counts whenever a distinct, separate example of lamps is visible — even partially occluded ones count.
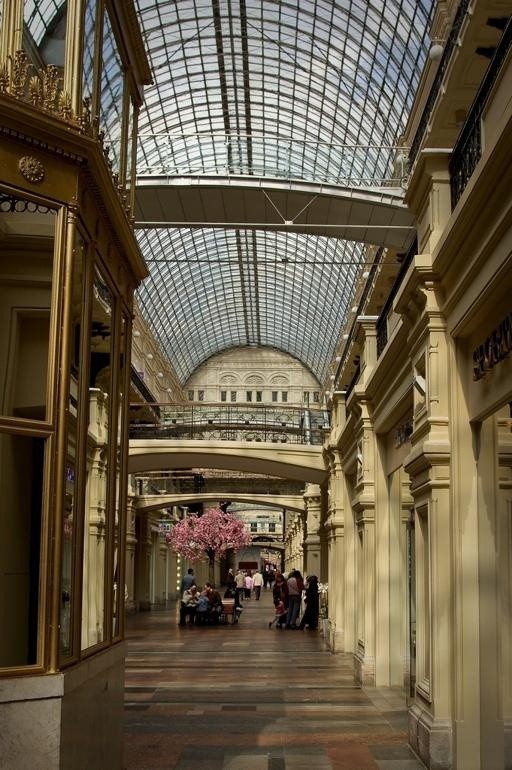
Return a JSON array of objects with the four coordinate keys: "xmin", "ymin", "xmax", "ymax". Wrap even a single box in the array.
[
  {"xmin": 395, "ymin": 154, "xmax": 410, "ymax": 167},
  {"xmin": 133, "ymin": 330, "xmax": 172, "ymax": 395},
  {"xmin": 429, "ymin": 39, "xmax": 448, "ymax": 61}
]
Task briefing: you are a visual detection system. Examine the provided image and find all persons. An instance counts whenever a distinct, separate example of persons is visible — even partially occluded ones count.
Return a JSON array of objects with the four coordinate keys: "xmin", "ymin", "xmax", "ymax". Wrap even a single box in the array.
[
  {"xmin": 223, "ymin": 566, "xmax": 296, "ymax": 600},
  {"xmin": 296, "ymin": 574, "xmax": 320, "ymax": 631},
  {"xmin": 273, "ymin": 572, "xmax": 290, "ymax": 624},
  {"xmin": 202, "ymin": 583, "xmax": 225, "ymax": 627},
  {"xmin": 195, "ymin": 591, "xmax": 210, "ymax": 626},
  {"xmin": 224, "ymin": 581, "xmax": 243, "ymax": 623},
  {"xmin": 285, "ymin": 570, "xmax": 299, "ymax": 630},
  {"xmin": 268, "ymin": 598, "xmax": 289, "ymax": 630},
  {"xmin": 295, "ymin": 571, "xmax": 306, "ymax": 618},
  {"xmin": 182, "ymin": 568, "xmax": 196, "ymax": 595},
  {"xmin": 178, "ymin": 584, "xmax": 201, "ymax": 624}
]
